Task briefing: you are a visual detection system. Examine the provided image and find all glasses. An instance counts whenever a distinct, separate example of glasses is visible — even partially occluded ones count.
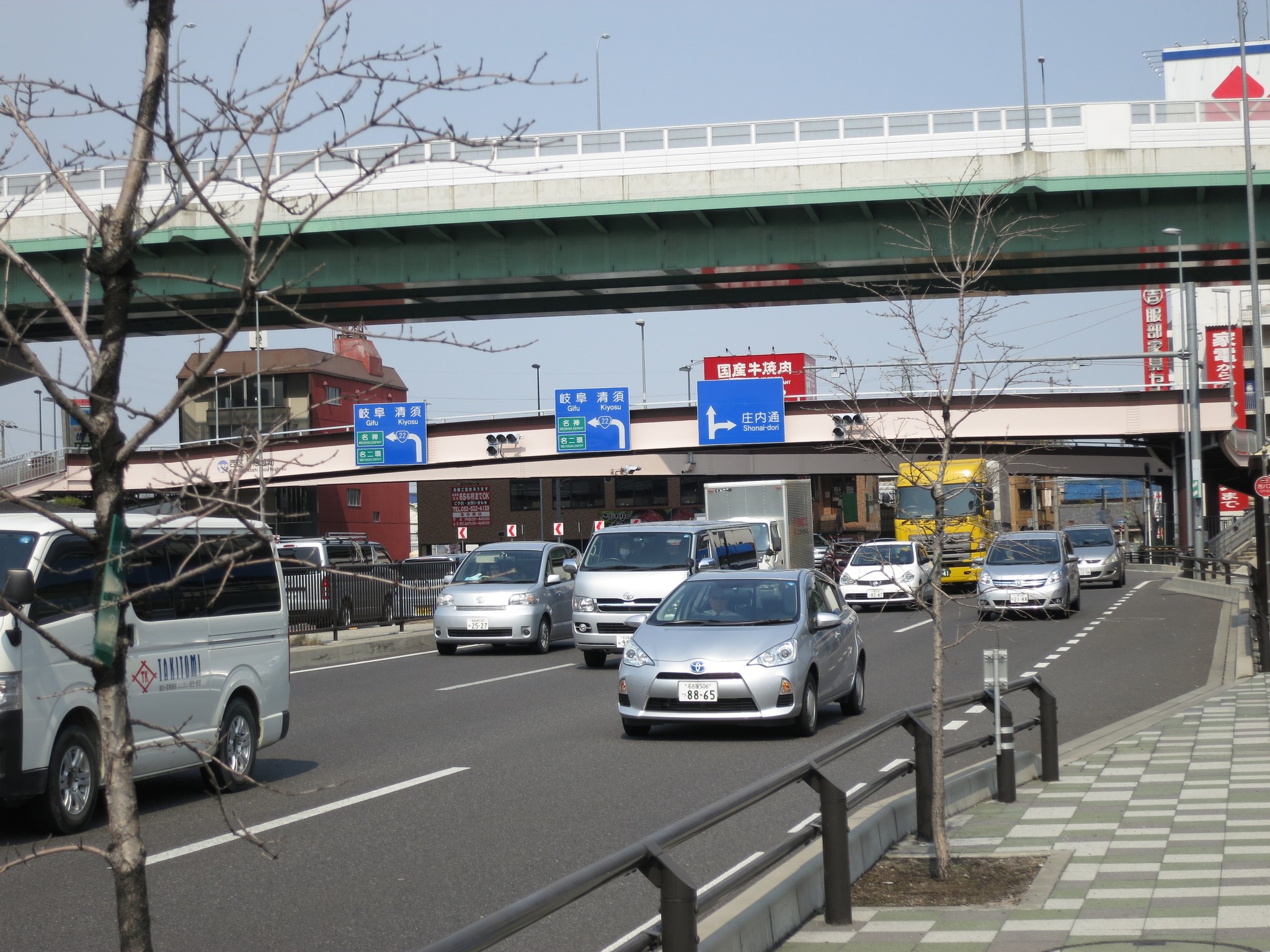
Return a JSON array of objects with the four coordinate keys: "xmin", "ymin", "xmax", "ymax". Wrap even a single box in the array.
[{"xmin": 710, "ymin": 595, "xmax": 727, "ymax": 600}]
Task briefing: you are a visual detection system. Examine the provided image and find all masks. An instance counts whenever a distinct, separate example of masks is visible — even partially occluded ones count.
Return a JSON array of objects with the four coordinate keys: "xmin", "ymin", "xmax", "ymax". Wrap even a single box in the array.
[
  {"xmin": 873, "ymin": 555, "xmax": 879, "ymax": 559},
  {"xmin": 900, "ymin": 556, "xmax": 907, "ymax": 562},
  {"xmin": 1006, "ymin": 550, "xmax": 1013, "ymax": 555},
  {"xmin": 619, "ymin": 548, "xmax": 630, "ymax": 556}
]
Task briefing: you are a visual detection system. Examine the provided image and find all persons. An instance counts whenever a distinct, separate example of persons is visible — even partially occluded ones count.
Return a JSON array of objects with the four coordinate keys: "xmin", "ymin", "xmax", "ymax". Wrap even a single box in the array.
[
  {"xmin": 1007, "ymin": 554, "xmax": 1015, "ymax": 560},
  {"xmin": 865, "ymin": 550, "xmax": 912, "ymax": 564},
  {"xmin": 490, "ymin": 563, "xmax": 500, "ymax": 576},
  {"xmin": 704, "ymin": 585, "xmax": 740, "ymax": 616},
  {"xmin": 612, "ymin": 537, "xmax": 639, "ymax": 564}
]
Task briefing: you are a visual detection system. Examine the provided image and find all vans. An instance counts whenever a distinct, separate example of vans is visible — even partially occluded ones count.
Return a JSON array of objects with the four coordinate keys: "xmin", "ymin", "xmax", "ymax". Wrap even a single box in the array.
[
  {"xmin": 561, "ymin": 521, "xmax": 759, "ymax": 669},
  {"xmin": 0, "ymin": 512, "xmax": 291, "ymax": 838},
  {"xmin": 398, "ymin": 552, "xmax": 470, "ymax": 618},
  {"xmin": 273, "ymin": 532, "xmax": 396, "ymax": 631}
]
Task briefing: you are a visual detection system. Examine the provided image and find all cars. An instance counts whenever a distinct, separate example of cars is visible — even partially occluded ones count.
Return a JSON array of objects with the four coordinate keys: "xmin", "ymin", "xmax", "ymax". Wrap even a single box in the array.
[
  {"xmin": 1060, "ymin": 524, "xmax": 1128, "ymax": 588},
  {"xmin": 972, "ymin": 530, "xmax": 1081, "ymax": 621},
  {"xmin": 839, "ymin": 538, "xmax": 934, "ymax": 612},
  {"xmin": 814, "ymin": 540, "xmax": 863, "ymax": 584},
  {"xmin": 433, "ymin": 540, "xmax": 584, "ymax": 655},
  {"xmin": 618, "ymin": 569, "xmax": 867, "ymax": 738}
]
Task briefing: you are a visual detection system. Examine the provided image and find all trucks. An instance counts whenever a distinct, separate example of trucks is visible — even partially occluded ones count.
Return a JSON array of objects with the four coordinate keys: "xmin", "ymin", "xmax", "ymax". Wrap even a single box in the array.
[
  {"xmin": 703, "ymin": 479, "xmax": 816, "ymax": 571},
  {"xmin": 881, "ymin": 458, "xmax": 1012, "ymax": 594}
]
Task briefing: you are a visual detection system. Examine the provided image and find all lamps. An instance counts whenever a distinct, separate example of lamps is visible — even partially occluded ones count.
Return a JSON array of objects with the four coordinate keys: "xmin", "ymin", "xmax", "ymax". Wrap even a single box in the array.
[
  {"xmin": 681, "ymin": 462, "xmax": 696, "ymax": 473},
  {"xmin": 1174, "ymin": 41, "xmax": 1182, "ymax": 47},
  {"xmin": 685, "ymin": 360, "xmax": 704, "ymax": 368},
  {"xmin": 387, "ymin": 394, "xmax": 392, "ymax": 398},
  {"xmin": 1232, "ymin": 37, "xmax": 1237, "ymax": 42},
  {"xmin": 772, "ymin": 346, "xmax": 776, "ymax": 355},
  {"xmin": 355, "ymin": 389, "xmax": 360, "ymax": 394},
  {"xmin": 323, "ymin": 381, "xmax": 328, "ymax": 386},
  {"xmin": 1203, "ymin": 38, "xmax": 1209, "ymax": 45},
  {"xmin": 726, "ymin": 348, "xmax": 737, "ymax": 356},
  {"xmin": 1141, "ymin": 50, "xmax": 1164, "ymax": 77},
  {"xmin": 806, "ymin": 354, "xmax": 837, "ymax": 362},
  {"xmin": 1260, "ymin": 35, "xmax": 1265, "ymax": 40},
  {"xmin": 747, "ymin": 346, "xmax": 752, "ymax": 356}
]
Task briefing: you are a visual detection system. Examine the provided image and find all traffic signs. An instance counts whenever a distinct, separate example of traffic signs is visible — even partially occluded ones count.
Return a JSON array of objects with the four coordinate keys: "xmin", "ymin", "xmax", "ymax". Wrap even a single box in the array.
[
  {"xmin": 554, "ymin": 387, "xmax": 632, "ymax": 453},
  {"xmin": 354, "ymin": 401, "xmax": 427, "ymax": 466},
  {"xmin": 697, "ymin": 378, "xmax": 786, "ymax": 445}
]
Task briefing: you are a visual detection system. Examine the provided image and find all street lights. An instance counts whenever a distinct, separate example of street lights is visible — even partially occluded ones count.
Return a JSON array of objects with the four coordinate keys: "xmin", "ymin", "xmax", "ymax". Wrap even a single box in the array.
[
  {"xmin": 1038, "ymin": 56, "xmax": 1047, "ymax": 106},
  {"xmin": 175, "ymin": 22, "xmax": 197, "ymax": 205},
  {"xmin": 596, "ymin": 34, "xmax": 612, "ymax": 131},
  {"xmin": 214, "ymin": 368, "xmax": 226, "ymax": 444},
  {"xmin": 532, "ymin": 364, "xmax": 540, "ymax": 416},
  {"xmin": 254, "ymin": 290, "xmax": 270, "ymax": 523},
  {"xmin": 1162, "ymin": 227, "xmax": 1204, "ymax": 581},
  {"xmin": 1212, "ymin": 288, "xmax": 1235, "ymax": 416},
  {"xmin": 636, "ymin": 318, "xmax": 646, "ymax": 408},
  {"xmin": 43, "ymin": 397, "xmax": 56, "ymax": 461},
  {"xmin": 34, "ymin": 389, "xmax": 43, "ymax": 454}
]
